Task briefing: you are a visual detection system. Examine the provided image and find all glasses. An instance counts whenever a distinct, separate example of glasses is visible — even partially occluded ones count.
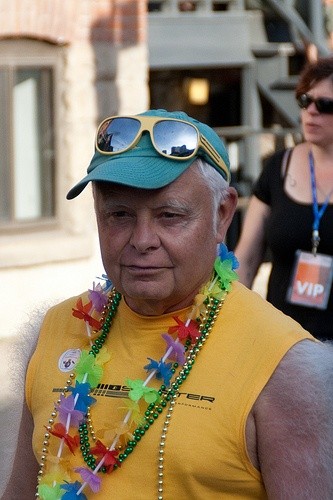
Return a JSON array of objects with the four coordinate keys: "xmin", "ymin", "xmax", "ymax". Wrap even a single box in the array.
[
  {"xmin": 296, "ymin": 93, "xmax": 333, "ymax": 114},
  {"xmin": 94, "ymin": 115, "xmax": 231, "ymax": 184}
]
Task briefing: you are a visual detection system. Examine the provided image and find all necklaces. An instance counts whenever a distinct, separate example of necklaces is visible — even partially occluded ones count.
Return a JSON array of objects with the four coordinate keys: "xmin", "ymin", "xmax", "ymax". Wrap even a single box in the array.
[{"xmin": 30, "ymin": 258, "xmax": 234, "ymax": 500}]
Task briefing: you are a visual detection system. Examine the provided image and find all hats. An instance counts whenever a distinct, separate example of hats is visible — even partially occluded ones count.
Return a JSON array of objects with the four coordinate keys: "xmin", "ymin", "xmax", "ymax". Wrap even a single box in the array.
[{"xmin": 66, "ymin": 109, "xmax": 231, "ymax": 201}]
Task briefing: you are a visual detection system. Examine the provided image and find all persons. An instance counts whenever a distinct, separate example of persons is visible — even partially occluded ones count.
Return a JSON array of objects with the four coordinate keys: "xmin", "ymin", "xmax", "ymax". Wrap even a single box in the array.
[
  {"xmin": 230, "ymin": 55, "xmax": 333, "ymax": 339},
  {"xmin": 2, "ymin": 111, "xmax": 333, "ymax": 500}
]
